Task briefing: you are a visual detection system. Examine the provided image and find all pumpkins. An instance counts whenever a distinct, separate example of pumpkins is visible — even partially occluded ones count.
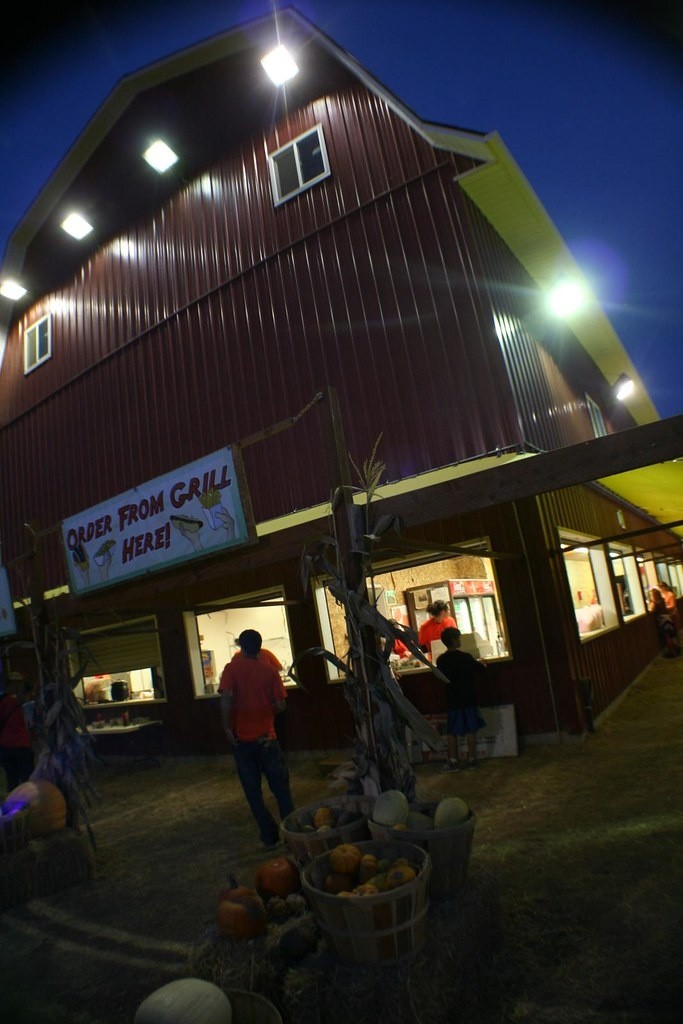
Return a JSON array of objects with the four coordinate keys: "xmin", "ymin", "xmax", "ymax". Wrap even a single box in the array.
[
  {"xmin": 6, "ymin": 778, "xmax": 67, "ymax": 837},
  {"xmin": 214, "ymin": 789, "xmax": 470, "ymax": 938},
  {"xmin": 133, "ymin": 978, "xmax": 232, "ymax": 1024}
]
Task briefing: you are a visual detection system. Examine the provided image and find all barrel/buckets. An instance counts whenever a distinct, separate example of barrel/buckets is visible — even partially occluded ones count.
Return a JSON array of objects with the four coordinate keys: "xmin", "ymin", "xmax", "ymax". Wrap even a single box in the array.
[
  {"xmin": 0, "ymin": 801, "xmax": 31, "ymax": 851},
  {"xmin": 301, "ymin": 841, "xmax": 432, "ymax": 968},
  {"xmin": 221, "ymin": 990, "xmax": 282, "ymax": 1024},
  {"xmin": 367, "ymin": 801, "xmax": 477, "ymax": 895},
  {"xmin": 280, "ymin": 794, "xmax": 370, "ymax": 875}
]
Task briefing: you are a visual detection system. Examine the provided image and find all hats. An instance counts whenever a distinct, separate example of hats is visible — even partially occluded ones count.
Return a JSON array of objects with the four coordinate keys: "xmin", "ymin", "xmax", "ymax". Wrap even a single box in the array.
[{"xmin": 236, "ymin": 630, "xmax": 261, "ymax": 650}]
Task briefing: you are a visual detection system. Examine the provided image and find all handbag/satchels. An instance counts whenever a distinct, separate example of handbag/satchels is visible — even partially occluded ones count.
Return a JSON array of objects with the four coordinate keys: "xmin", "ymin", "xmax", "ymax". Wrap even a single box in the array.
[{"xmin": 657, "ymin": 614, "xmax": 674, "ymax": 631}]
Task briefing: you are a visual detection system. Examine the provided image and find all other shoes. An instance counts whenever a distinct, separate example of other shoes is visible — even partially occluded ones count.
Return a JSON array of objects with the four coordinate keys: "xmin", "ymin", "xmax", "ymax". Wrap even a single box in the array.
[
  {"xmin": 662, "ymin": 647, "xmax": 680, "ymax": 658},
  {"xmin": 255, "ymin": 843, "xmax": 280, "ymax": 859},
  {"xmin": 467, "ymin": 756, "xmax": 479, "ymax": 769},
  {"xmin": 439, "ymin": 759, "xmax": 460, "ymax": 773}
]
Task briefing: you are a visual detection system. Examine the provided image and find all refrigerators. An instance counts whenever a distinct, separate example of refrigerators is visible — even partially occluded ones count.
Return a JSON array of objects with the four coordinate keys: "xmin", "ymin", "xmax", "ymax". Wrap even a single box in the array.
[{"xmin": 406, "ymin": 579, "xmax": 501, "ymax": 657}]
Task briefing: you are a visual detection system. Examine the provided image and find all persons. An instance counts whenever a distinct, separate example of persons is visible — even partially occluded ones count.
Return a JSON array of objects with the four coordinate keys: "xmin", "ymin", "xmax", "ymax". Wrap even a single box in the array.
[
  {"xmin": 418, "ymin": 601, "xmax": 458, "ymax": 652},
  {"xmin": 436, "ymin": 627, "xmax": 487, "ymax": 773},
  {"xmin": 388, "ymin": 619, "xmax": 414, "ymax": 659},
  {"xmin": 219, "ymin": 630, "xmax": 296, "ymax": 844},
  {"xmin": 648, "ymin": 582, "xmax": 682, "ymax": 659},
  {"xmin": 1, "ymin": 671, "xmax": 36, "ymax": 791}
]
